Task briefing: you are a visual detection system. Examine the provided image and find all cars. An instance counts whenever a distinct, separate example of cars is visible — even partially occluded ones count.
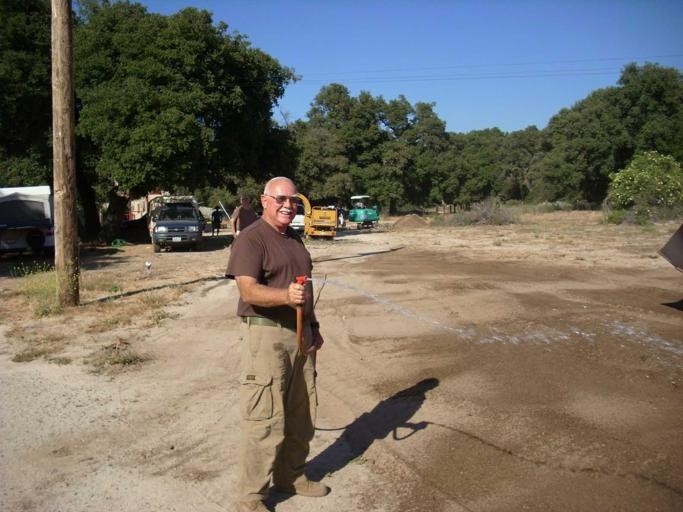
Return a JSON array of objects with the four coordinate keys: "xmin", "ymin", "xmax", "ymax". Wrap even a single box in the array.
[{"xmin": 153, "ymin": 206, "xmax": 203, "ymax": 253}]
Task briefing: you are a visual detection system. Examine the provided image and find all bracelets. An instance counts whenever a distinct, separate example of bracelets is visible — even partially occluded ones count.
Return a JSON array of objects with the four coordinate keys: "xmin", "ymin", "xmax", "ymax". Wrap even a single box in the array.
[{"xmin": 310, "ymin": 322, "xmax": 319, "ymax": 329}]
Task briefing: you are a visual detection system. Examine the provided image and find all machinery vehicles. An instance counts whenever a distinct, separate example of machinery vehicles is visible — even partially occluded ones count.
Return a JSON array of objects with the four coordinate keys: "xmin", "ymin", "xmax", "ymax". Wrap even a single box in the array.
[{"xmin": 297, "ymin": 194, "xmax": 379, "ymax": 240}]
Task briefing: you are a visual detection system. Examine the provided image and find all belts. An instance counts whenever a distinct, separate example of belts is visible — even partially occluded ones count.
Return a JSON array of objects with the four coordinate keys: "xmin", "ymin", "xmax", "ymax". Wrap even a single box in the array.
[{"xmin": 241, "ymin": 314, "xmax": 312, "ymax": 328}]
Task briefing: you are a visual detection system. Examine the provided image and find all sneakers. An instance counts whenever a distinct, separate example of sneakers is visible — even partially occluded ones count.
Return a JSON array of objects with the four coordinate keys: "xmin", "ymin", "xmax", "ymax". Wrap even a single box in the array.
[
  {"xmin": 235, "ymin": 497, "xmax": 272, "ymax": 512},
  {"xmin": 273, "ymin": 479, "xmax": 330, "ymax": 498}
]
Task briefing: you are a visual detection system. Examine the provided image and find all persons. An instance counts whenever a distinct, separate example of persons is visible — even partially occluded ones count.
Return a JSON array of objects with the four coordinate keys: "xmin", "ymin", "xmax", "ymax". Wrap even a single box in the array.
[
  {"xmin": 338, "ymin": 207, "xmax": 344, "ymax": 225},
  {"xmin": 230, "ymin": 195, "xmax": 257, "ymax": 238},
  {"xmin": 224, "ymin": 177, "xmax": 329, "ymax": 510},
  {"xmin": 209, "ymin": 206, "xmax": 223, "ymax": 237}
]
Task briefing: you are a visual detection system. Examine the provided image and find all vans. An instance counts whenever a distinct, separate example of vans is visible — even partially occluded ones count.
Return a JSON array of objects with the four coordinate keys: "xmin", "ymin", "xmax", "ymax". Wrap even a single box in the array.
[{"xmin": 0, "ymin": 185, "xmax": 54, "ymax": 257}]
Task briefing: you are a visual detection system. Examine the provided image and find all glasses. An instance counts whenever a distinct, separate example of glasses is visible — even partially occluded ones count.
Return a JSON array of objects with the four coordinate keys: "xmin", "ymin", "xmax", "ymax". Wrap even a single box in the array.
[{"xmin": 263, "ymin": 193, "xmax": 300, "ymax": 204}]
